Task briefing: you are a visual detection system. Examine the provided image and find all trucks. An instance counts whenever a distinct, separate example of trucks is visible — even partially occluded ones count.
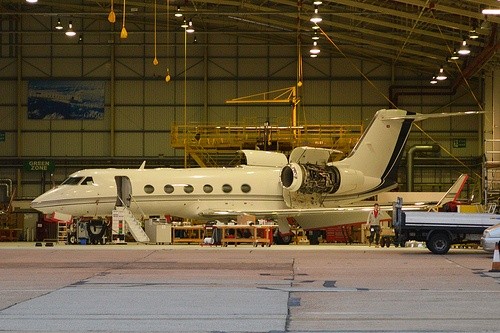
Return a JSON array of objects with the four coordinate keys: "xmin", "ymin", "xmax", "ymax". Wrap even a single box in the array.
[{"xmin": 391, "ymin": 197, "xmax": 500, "ymax": 255}]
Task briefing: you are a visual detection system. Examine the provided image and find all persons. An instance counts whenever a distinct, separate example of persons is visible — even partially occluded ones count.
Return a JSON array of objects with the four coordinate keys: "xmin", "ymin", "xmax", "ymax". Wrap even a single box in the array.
[{"xmin": 365, "ymin": 202, "xmax": 381, "ymax": 248}]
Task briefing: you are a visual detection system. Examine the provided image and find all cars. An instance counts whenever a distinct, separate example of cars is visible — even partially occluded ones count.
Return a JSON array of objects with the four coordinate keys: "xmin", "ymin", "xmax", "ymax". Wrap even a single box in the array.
[{"xmin": 481, "ymin": 223, "xmax": 500, "ymax": 251}]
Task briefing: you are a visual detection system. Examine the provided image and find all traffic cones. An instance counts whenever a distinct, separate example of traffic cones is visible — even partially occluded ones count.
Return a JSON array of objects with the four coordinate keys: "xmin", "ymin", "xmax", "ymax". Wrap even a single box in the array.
[{"xmin": 488, "ymin": 242, "xmax": 500, "ymax": 273}]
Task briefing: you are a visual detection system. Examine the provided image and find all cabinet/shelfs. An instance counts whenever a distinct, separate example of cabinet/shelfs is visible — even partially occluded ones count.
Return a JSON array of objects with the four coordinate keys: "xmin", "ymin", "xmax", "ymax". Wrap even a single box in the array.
[
  {"xmin": 290, "ymin": 228, "xmax": 310, "ymax": 245},
  {"xmin": 213, "ymin": 225, "xmax": 279, "ymax": 248},
  {"xmin": 171, "ymin": 226, "xmax": 201, "ymax": 244}
]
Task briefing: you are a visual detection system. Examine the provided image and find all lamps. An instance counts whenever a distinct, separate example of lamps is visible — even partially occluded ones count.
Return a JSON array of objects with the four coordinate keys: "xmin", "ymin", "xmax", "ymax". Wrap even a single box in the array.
[
  {"xmin": 55, "ymin": 16, "xmax": 63, "ymax": 30},
  {"xmin": 64, "ymin": 20, "xmax": 77, "ymax": 37},
  {"xmin": 152, "ymin": 0, "xmax": 159, "ymax": 65},
  {"xmin": 165, "ymin": 0, "xmax": 171, "ymax": 82},
  {"xmin": 308, "ymin": 0, "xmax": 322, "ymax": 57},
  {"xmin": 108, "ymin": 0, "xmax": 116, "ymax": 23},
  {"xmin": 430, "ymin": 6, "xmax": 500, "ymax": 84},
  {"xmin": 297, "ymin": 39, "xmax": 302, "ymax": 87},
  {"xmin": 174, "ymin": 5, "xmax": 196, "ymax": 44},
  {"xmin": 120, "ymin": 0, "xmax": 128, "ymax": 38}
]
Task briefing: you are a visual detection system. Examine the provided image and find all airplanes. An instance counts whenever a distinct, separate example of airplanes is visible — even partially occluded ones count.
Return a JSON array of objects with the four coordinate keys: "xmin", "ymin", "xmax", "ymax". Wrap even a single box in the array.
[{"xmin": 30, "ymin": 108, "xmax": 472, "ymax": 245}]
